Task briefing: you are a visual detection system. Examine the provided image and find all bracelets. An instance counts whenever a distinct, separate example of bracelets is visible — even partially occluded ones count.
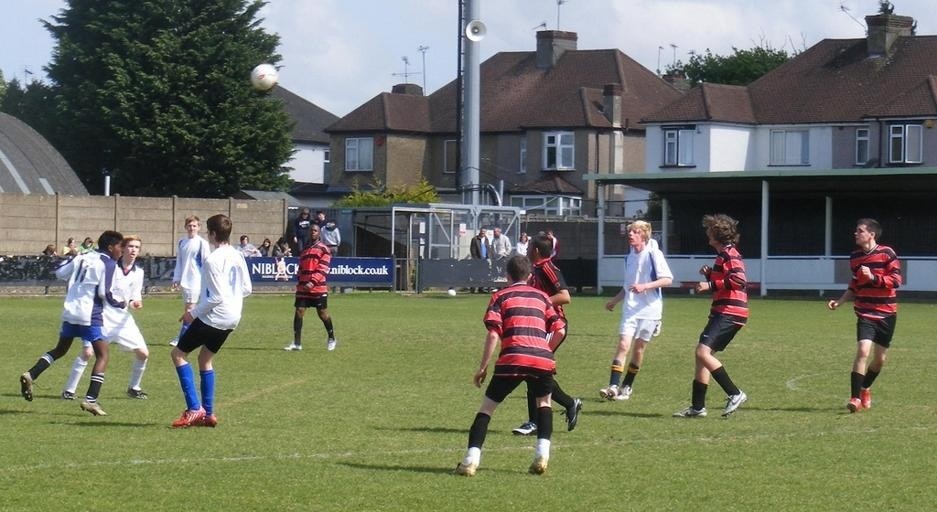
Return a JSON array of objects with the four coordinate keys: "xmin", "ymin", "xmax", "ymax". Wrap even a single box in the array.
[{"xmin": 707, "ymin": 281, "xmax": 711, "ymax": 290}]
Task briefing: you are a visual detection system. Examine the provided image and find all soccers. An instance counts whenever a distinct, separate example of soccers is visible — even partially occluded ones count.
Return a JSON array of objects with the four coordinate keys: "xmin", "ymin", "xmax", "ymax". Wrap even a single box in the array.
[
  {"xmin": 250, "ymin": 63, "xmax": 277, "ymax": 90},
  {"xmin": 447, "ymin": 287, "xmax": 457, "ymax": 297}
]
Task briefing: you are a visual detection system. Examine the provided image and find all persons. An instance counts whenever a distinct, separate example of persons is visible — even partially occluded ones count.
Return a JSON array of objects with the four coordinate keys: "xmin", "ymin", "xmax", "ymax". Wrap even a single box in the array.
[
  {"xmin": 61, "ymin": 235, "xmax": 149, "ymax": 401},
  {"xmin": 41, "ymin": 237, "xmax": 95, "ymax": 255},
  {"xmin": 511, "ymin": 232, "xmax": 582, "ymax": 436},
  {"xmin": 283, "ymin": 223, "xmax": 337, "ymax": 351},
  {"xmin": 672, "ymin": 214, "xmax": 750, "ymax": 417},
  {"xmin": 458, "ymin": 222, "xmax": 560, "ymax": 295},
  {"xmin": 235, "ymin": 206, "xmax": 341, "ymax": 258},
  {"xmin": 826, "ymin": 218, "xmax": 904, "ymax": 413},
  {"xmin": 170, "ymin": 213, "xmax": 253, "ymax": 425},
  {"xmin": 456, "ymin": 255, "xmax": 567, "ymax": 475},
  {"xmin": 170, "ymin": 215, "xmax": 211, "ymax": 346},
  {"xmin": 20, "ymin": 230, "xmax": 128, "ymax": 416},
  {"xmin": 598, "ymin": 220, "xmax": 673, "ymax": 400}
]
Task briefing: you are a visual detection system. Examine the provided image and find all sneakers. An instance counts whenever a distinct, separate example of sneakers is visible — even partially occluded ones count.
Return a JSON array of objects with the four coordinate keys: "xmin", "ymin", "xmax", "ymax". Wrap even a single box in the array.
[
  {"xmin": 722, "ymin": 388, "xmax": 747, "ymax": 416},
  {"xmin": 614, "ymin": 385, "xmax": 632, "ymax": 400},
  {"xmin": 173, "ymin": 406, "xmax": 206, "ymax": 430},
  {"xmin": 847, "ymin": 398, "xmax": 862, "ymax": 413},
  {"xmin": 456, "ymin": 460, "xmax": 477, "ymax": 476},
  {"xmin": 20, "ymin": 372, "xmax": 108, "ymax": 417},
  {"xmin": 599, "ymin": 386, "xmax": 619, "ymax": 398},
  {"xmin": 170, "ymin": 335, "xmax": 178, "ymax": 346},
  {"xmin": 127, "ymin": 385, "xmax": 148, "ymax": 400},
  {"xmin": 201, "ymin": 409, "xmax": 216, "ymax": 427},
  {"xmin": 511, "ymin": 397, "xmax": 583, "ymax": 475},
  {"xmin": 284, "ymin": 342, "xmax": 302, "ymax": 351},
  {"xmin": 327, "ymin": 337, "xmax": 337, "ymax": 351},
  {"xmin": 673, "ymin": 406, "xmax": 708, "ymax": 418},
  {"xmin": 859, "ymin": 388, "xmax": 871, "ymax": 408}
]
